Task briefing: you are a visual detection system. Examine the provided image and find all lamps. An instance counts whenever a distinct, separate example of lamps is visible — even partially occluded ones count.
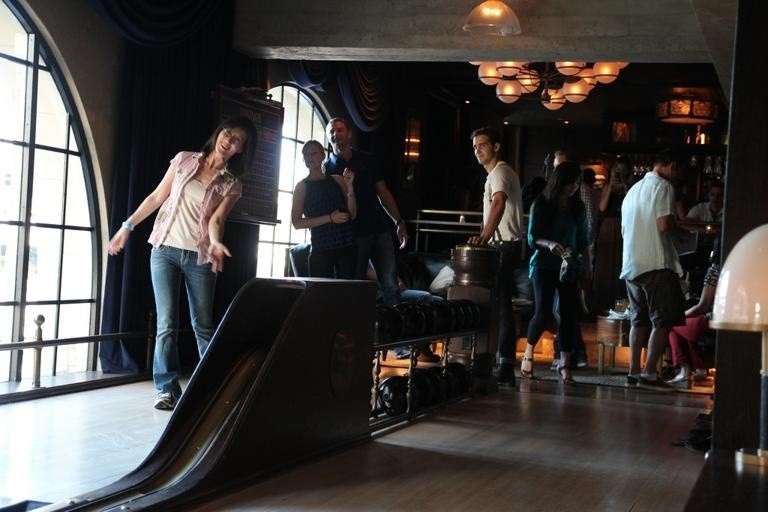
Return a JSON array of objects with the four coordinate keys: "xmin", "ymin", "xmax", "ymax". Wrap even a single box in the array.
[
  {"xmin": 709, "ymin": 224, "xmax": 767, "ymax": 474},
  {"xmin": 463, "ymin": 0, "xmax": 715, "ymax": 123}
]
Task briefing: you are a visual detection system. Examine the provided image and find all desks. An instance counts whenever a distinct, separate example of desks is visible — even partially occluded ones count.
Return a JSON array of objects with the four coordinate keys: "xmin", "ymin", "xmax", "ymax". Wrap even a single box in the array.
[{"xmin": 596, "ymin": 316, "xmax": 630, "ymax": 374}]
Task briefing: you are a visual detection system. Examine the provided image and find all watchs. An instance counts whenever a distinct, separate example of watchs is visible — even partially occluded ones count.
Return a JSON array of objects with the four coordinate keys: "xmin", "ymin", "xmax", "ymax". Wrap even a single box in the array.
[{"xmin": 397, "ymin": 218, "xmax": 405, "ymax": 225}]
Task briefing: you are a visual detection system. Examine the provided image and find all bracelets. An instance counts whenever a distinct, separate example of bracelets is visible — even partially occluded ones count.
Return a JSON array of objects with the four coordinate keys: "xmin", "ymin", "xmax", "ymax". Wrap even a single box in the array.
[
  {"xmin": 328, "ymin": 214, "xmax": 333, "ymax": 224},
  {"xmin": 345, "ymin": 191, "xmax": 356, "ymax": 198},
  {"xmin": 121, "ymin": 222, "xmax": 135, "ymax": 231}
]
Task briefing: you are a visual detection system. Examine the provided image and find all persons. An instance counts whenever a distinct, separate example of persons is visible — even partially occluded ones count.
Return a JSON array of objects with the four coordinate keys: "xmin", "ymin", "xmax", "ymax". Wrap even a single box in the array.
[
  {"xmin": 324, "ymin": 116, "xmax": 416, "ymax": 359},
  {"xmin": 467, "ymin": 128, "xmax": 523, "ymax": 388},
  {"xmin": 107, "ymin": 116, "xmax": 256, "ymax": 409},
  {"xmin": 292, "ymin": 140, "xmax": 358, "ymax": 280},
  {"xmin": 523, "ymin": 143, "xmax": 725, "ymax": 393}
]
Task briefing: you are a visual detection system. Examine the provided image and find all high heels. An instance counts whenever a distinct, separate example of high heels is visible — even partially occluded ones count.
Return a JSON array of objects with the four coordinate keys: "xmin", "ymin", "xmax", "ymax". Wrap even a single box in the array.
[
  {"xmin": 520, "ymin": 355, "xmax": 535, "ymax": 380},
  {"xmin": 557, "ymin": 359, "xmax": 578, "ymax": 386}
]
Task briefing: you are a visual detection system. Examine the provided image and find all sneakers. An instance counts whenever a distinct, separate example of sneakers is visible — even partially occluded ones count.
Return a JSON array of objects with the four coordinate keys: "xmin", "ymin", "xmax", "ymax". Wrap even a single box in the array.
[
  {"xmin": 152, "ymin": 392, "xmax": 180, "ymax": 411},
  {"xmin": 625, "ymin": 369, "xmax": 707, "ymax": 393},
  {"xmin": 550, "ymin": 359, "xmax": 588, "ymax": 372},
  {"xmin": 496, "ymin": 363, "xmax": 515, "ymax": 384}
]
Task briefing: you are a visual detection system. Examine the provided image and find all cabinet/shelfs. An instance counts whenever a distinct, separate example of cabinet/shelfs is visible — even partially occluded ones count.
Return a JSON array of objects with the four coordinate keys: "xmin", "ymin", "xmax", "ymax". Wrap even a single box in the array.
[{"xmin": 369, "ymin": 285, "xmax": 492, "ymax": 431}]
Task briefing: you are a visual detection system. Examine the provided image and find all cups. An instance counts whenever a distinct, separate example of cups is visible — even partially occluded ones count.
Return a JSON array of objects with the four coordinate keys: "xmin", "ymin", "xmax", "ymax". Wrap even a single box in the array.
[
  {"xmin": 614, "ymin": 298, "xmax": 629, "ymax": 312},
  {"xmin": 733, "ymin": 447, "xmax": 768, "ymax": 491}
]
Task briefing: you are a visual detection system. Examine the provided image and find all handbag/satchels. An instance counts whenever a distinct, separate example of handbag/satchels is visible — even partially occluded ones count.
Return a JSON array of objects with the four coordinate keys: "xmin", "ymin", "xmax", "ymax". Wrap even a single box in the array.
[{"xmin": 557, "ymin": 254, "xmax": 594, "ymax": 285}]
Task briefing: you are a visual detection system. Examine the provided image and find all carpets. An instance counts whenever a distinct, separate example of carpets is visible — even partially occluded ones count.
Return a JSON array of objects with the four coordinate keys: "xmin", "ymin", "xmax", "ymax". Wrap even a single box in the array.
[{"xmin": 373, "ymin": 350, "xmax": 716, "ymax": 394}]
